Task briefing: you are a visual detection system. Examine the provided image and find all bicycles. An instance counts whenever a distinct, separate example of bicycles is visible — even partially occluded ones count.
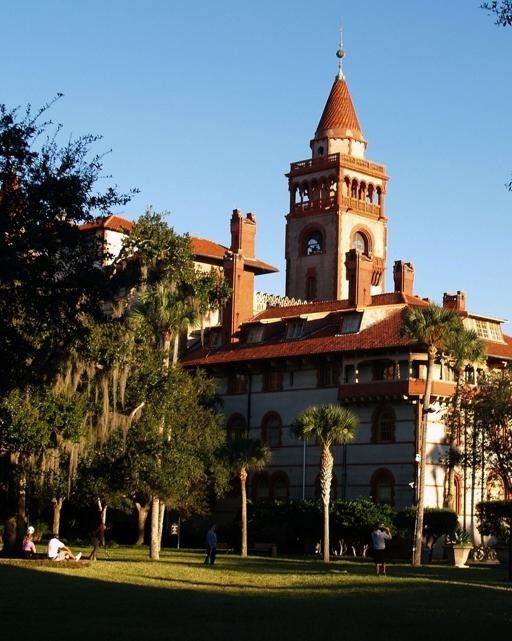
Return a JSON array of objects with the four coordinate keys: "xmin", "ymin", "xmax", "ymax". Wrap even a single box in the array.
[{"xmin": 472, "ymin": 545, "xmax": 497, "ymax": 562}]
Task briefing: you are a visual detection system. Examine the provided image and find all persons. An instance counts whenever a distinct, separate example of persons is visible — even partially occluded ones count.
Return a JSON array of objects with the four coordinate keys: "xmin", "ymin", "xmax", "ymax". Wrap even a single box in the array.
[
  {"xmin": 371, "ymin": 523, "xmax": 392, "ymax": 576},
  {"xmin": 87, "ymin": 510, "xmax": 111, "ymax": 561},
  {"xmin": 203, "ymin": 522, "xmax": 218, "ymax": 565},
  {"xmin": 47, "ymin": 533, "xmax": 82, "ymax": 562},
  {"xmin": 22, "ymin": 531, "xmax": 44, "ymax": 559}
]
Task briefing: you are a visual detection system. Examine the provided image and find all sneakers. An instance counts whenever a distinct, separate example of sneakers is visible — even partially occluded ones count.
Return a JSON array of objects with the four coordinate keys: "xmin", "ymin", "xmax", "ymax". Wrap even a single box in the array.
[{"xmin": 74, "ymin": 551, "xmax": 82, "ymax": 562}]
